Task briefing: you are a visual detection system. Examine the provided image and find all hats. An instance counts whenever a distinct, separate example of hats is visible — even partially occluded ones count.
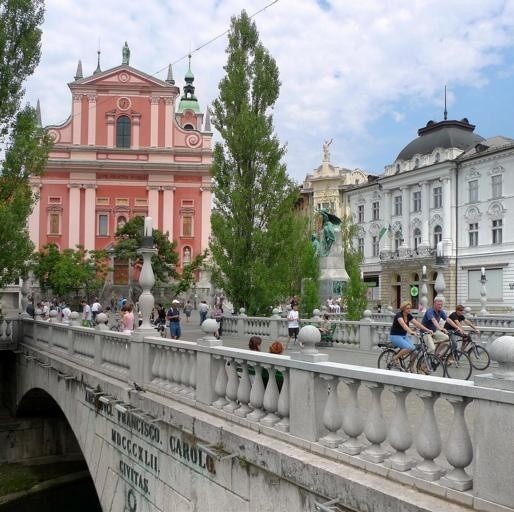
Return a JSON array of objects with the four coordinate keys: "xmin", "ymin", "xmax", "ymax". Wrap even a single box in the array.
[{"xmin": 172, "ymin": 300, "xmax": 180, "ymax": 304}]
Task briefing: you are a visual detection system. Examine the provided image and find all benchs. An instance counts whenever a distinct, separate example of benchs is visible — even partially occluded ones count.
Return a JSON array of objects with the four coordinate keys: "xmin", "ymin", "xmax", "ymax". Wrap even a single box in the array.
[
  {"xmin": 227, "ymin": 361, "xmax": 283, "ymax": 394},
  {"xmin": 315, "ymin": 323, "xmax": 337, "ymax": 347}
]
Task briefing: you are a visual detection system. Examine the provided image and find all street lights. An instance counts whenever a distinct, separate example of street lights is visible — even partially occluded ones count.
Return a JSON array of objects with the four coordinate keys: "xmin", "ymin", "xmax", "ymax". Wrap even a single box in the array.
[
  {"xmin": 477, "ymin": 266, "xmax": 490, "ymax": 317},
  {"xmin": 420, "ymin": 263, "xmax": 428, "ymax": 314}
]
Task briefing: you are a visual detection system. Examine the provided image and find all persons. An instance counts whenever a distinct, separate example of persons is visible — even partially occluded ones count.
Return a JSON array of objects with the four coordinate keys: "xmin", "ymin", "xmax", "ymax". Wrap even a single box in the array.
[
  {"xmin": 323, "ymin": 138, "xmax": 333, "ymax": 162},
  {"xmin": 247, "ymin": 336, "xmax": 262, "ymax": 352},
  {"xmin": 390, "ymin": 301, "xmax": 432, "ymax": 374},
  {"xmin": 318, "ymin": 313, "xmax": 330, "ymax": 335},
  {"xmin": 26, "ymin": 297, "xmax": 223, "ymax": 340},
  {"xmin": 269, "ymin": 341, "xmax": 284, "ymax": 354},
  {"xmin": 443, "ymin": 304, "xmax": 480, "ymax": 361},
  {"xmin": 326, "ymin": 296, "xmax": 336, "ymax": 312},
  {"xmin": 419, "ymin": 294, "xmax": 463, "ymax": 377},
  {"xmin": 315, "ymin": 208, "xmax": 334, "ymax": 256},
  {"xmin": 334, "ymin": 296, "xmax": 340, "ymax": 312},
  {"xmin": 311, "ymin": 233, "xmax": 319, "ymax": 257},
  {"xmin": 286, "ymin": 302, "xmax": 300, "ymax": 349}
]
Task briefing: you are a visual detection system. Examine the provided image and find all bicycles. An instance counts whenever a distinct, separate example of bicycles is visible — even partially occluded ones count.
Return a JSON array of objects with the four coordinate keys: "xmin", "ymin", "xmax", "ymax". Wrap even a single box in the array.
[
  {"xmin": 374, "ymin": 330, "xmax": 491, "ymax": 383},
  {"xmin": 110, "ymin": 316, "xmax": 168, "ymax": 339}
]
